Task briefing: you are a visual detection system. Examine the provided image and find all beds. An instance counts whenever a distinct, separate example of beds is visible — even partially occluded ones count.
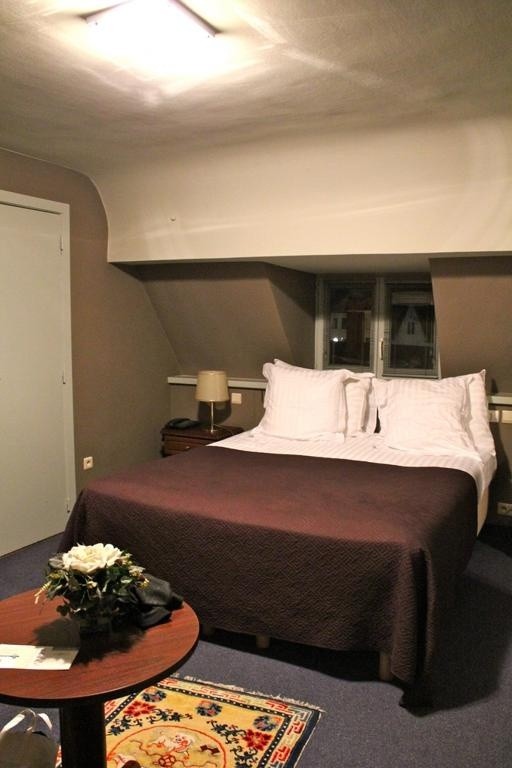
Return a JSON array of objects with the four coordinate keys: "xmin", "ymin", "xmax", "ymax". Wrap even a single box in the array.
[{"xmin": 57, "ymin": 363, "xmax": 498, "ymax": 690}]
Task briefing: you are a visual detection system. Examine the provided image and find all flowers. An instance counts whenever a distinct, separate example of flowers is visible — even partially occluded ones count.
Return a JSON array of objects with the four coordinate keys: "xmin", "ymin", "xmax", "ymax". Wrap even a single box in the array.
[{"xmin": 36, "ymin": 540, "xmax": 185, "ymax": 638}]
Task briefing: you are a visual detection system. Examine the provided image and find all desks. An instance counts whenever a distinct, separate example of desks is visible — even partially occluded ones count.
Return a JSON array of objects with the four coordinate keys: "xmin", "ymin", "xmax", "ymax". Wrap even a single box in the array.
[{"xmin": 0, "ymin": 589, "xmax": 200, "ymax": 768}]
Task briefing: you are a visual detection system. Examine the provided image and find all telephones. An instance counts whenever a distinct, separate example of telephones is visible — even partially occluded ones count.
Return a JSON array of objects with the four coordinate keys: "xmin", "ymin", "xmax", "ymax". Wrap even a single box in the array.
[{"xmin": 168, "ymin": 417, "xmax": 200, "ymax": 430}]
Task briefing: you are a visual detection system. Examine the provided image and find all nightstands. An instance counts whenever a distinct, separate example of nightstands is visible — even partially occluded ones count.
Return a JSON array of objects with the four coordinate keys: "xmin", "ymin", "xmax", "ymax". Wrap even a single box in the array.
[{"xmin": 161, "ymin": 422, "xmax": 243, "ymax": 457}]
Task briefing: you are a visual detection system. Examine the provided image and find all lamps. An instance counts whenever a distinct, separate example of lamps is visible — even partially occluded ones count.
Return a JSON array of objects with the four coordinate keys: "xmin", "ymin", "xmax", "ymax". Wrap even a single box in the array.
[
  {"xmin": 77, "ymin": 0, "xmax": 223, "ymax": 76},
  {"xmin": 193, "ymin": 369, "xmax": 231, "ymax": 433}
]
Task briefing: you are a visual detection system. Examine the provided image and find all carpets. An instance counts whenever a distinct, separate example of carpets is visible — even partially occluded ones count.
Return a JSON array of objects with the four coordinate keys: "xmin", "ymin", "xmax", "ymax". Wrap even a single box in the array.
[{"xmin": 54, "ymin": 673, "xmax": 321, "ymax": 767}]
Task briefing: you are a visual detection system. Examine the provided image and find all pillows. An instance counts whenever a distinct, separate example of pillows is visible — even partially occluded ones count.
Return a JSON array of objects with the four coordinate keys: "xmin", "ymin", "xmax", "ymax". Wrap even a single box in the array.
[
  {"xmin": 372, "ymin": 373, "xmax": 473, "ymax": 445},
  {"xmin": 261, "ymin": 361, "xmax": 357, "ymax": 440},
  {"xmin": 274, "ymin": 359, "xmax": 377, "ymax": 437}
]
